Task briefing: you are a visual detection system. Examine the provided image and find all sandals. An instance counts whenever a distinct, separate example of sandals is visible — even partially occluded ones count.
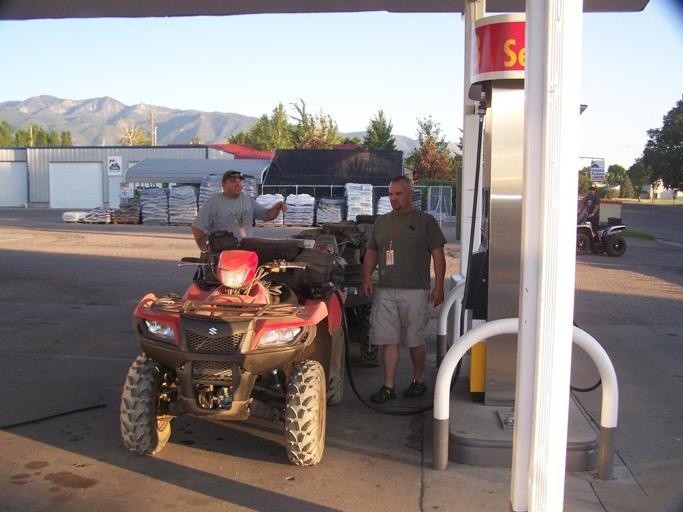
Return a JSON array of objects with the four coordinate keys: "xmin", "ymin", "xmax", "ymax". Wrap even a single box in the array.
[
  {"xmin": 403, "ymin": 380, "xmax": 428, "ymax": 398},
  {"xmin": 370, "ymin": 384, "xmax": 397, "ymax": 404}
]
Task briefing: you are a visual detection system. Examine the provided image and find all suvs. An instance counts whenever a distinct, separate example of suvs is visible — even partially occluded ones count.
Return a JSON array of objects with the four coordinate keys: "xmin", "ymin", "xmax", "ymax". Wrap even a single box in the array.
[{"xmin": 577, "ymin": 214, "xmax": 628, "ymax": 257}]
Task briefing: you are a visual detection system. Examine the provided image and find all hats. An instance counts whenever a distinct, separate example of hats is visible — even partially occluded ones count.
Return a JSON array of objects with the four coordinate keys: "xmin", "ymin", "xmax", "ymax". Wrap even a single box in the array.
[{"xmin": 222, "ymin": 170, "xmax": 245, "ymax": 182}]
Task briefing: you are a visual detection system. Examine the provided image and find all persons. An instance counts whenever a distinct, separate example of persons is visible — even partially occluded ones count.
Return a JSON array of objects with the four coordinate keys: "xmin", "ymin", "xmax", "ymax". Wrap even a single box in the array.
[
  {"xmin": 191, "ymin": 170, "xmax": 288, "ymax": 259},
  {"xmin": 578, "ymin": 187, "xmax": 600, "ymax": 236},
  {"xmin": 361, "ymin": 176, "xmax": 446, "ymax": 403}
]
